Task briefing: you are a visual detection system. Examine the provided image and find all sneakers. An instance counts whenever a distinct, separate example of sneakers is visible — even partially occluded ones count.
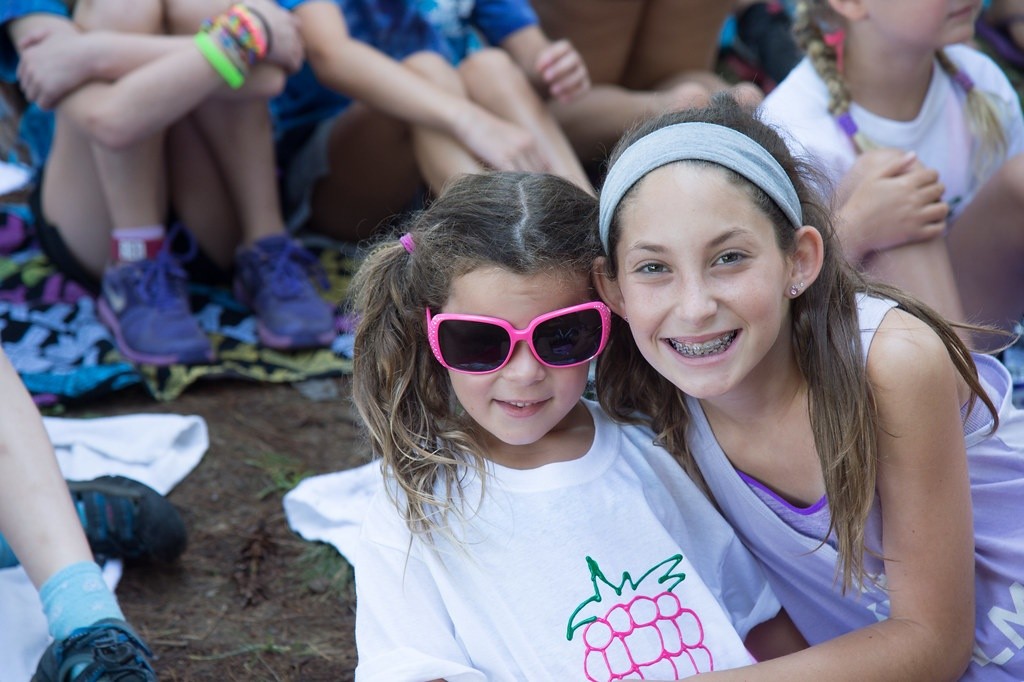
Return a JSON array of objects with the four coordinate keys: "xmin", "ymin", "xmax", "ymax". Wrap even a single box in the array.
[
  {"xmin": 30, "ymin": 618, "xmax": 157, "ymax": 682},
  {"xmin": 236, "ymin": 233, "xmax": 339, "ymax": 351},
  {"xmin": 64, "ymin": 474, "xmax": 187, "ymax": 571},
  {"xmin": 98, "ymin": 227, "xmax": 213, "ymax": 365}
]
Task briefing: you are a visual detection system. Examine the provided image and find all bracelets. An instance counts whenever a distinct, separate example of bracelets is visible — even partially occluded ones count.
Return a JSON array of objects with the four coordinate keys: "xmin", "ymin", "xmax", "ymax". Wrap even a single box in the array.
[{"xmin": 193, "ymin": 5, "xmax": 272, "ymax": 89}]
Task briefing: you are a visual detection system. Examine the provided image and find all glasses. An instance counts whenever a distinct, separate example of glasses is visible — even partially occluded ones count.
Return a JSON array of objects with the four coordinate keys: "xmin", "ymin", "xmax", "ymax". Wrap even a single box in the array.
[{"xmin": 425, "ymin": 300, "xmax": 611, "ymax": 375}]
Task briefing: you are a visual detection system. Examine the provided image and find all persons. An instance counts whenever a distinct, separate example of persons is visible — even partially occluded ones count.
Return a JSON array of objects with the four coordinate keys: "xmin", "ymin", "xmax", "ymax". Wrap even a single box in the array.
[
  {"xmin": 283, "ymin": 172, "xmax": 807, "ymax": 682},
  {"xmin": 278, "ymin": 0, "xmax": 1024, "ymax": 365},
  {"xmin": 1, "ymin": 342, "xmax": 189, "ymax": 682},
  {"xmin": 591, "ymin": 95, "xmax": 1024, "ymax": 682},
  {"xmin": 0, "ymin": 0, "xmax": 336, "ymax": 363}
]
